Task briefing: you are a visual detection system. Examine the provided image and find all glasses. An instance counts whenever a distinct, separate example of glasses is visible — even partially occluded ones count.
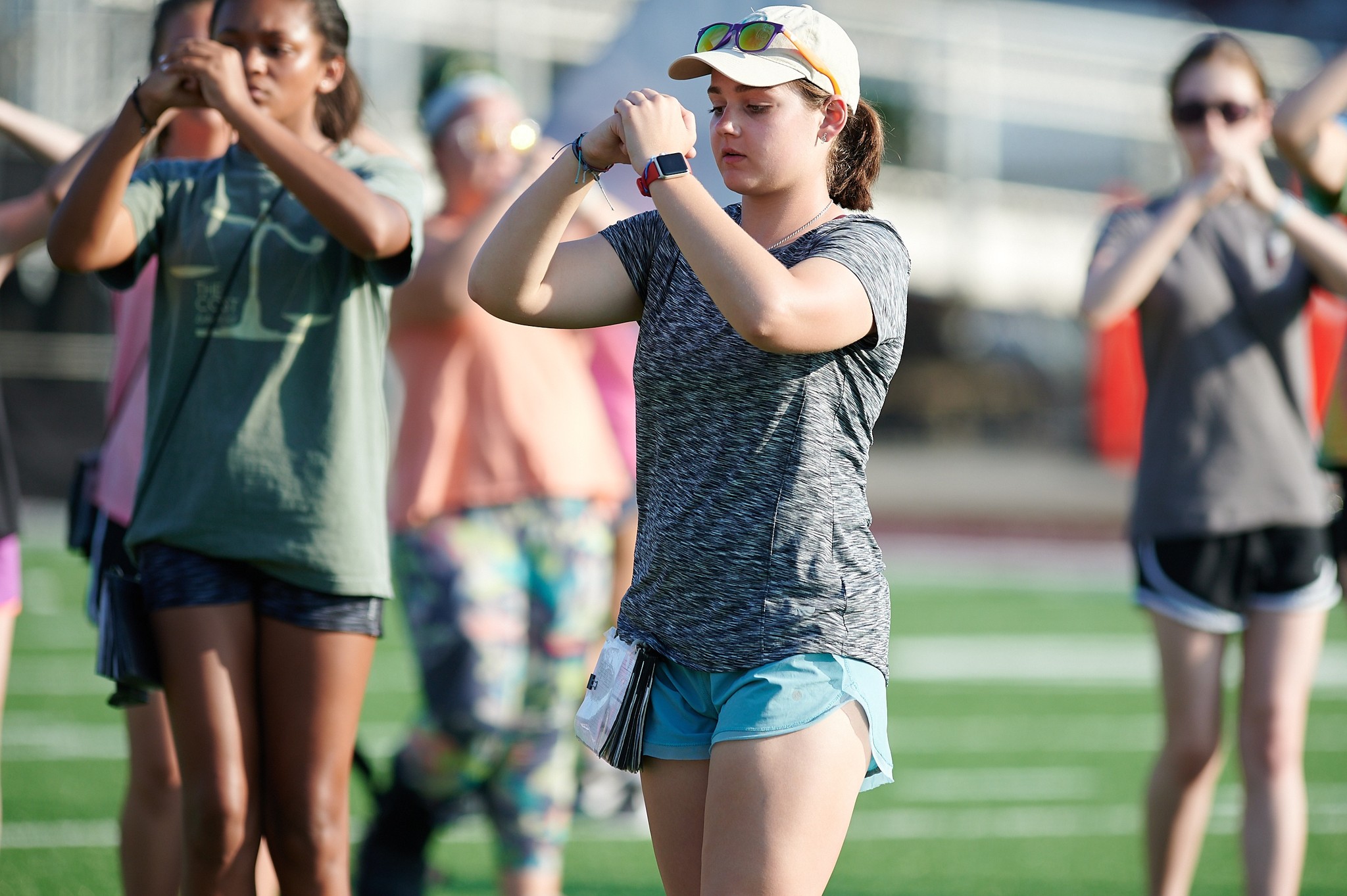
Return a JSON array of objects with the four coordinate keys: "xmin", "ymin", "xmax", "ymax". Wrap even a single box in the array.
[
  {"xmin": 694, "ymin": 21, "xmax": 843, "ymax": 97},
  {"xmin": 1176, "ymin": 100, "xmax": 1252, "ymax": 127}
]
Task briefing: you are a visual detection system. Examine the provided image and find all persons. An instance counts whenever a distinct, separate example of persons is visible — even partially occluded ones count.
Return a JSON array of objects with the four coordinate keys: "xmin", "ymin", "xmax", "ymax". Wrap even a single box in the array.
[
  {"xmin": 465, "ymin": 4, "xmax": 912, "ymax": 896},
  {"xmin": 0, "ymin": 0, "xmax": 224, "ymax": 896},
  {"xmin": 1078, "ymin": 31, "xmax": 1347, "ymax": 896},
  {"xmin": 43, "ymin": 0, "xmax": 427, "ymax": 896},
  {"xmin": 1270, "ymin": 49, "xmax": 1347, "ymax": 592},
  {"xmin": 352, "ymin": 46, "xmax": 656, "ymax": 896}
]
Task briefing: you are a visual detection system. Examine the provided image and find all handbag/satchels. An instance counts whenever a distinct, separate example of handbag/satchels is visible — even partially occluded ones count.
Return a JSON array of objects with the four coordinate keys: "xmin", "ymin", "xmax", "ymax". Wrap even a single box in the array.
[
  {"xmin": 94, "ymin": 569, "xmax": 168, "ymax": 690},
  {"xmin": 576, "ymin": 624, "xmax": 657, "ymax": 774},
  {"xmin": 67, "ymin": 450, "xmax": 100, "ymax": 553}
]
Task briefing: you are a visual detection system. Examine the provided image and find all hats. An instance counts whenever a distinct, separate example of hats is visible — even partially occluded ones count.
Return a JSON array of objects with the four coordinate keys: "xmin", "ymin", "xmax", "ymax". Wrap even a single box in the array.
[{"xmin": 668, "ymin": 5, "xmax": 861, "ymax": 117}]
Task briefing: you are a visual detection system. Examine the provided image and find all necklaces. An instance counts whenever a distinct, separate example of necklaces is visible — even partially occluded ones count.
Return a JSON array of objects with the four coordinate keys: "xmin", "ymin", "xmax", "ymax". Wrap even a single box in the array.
[{"xmin": 765, "ymin": 199, "xmax": 834, "ymax": 249}]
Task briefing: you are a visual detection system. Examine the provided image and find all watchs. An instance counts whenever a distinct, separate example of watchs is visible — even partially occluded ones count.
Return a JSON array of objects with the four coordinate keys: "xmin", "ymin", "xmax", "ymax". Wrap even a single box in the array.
[{"xmin": 636, "ymin": 152, "xmax": 692, "ymax": 198}]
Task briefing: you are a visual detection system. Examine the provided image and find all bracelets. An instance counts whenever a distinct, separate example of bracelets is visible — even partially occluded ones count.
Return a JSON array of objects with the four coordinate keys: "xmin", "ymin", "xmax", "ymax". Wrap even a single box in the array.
[
  {"xmin": 131, "ymin": 77, "xmax": 158, "ymax": 135},
  {"xmin": 551, "ymin": 132, "xmax": 615, "ymax": 211}
]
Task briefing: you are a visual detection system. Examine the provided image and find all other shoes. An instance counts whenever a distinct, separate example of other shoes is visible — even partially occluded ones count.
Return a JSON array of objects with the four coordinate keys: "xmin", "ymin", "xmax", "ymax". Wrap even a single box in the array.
[{"xmin": 570, "ymin": 756, "xmax": 651, "ymax": 835}]
